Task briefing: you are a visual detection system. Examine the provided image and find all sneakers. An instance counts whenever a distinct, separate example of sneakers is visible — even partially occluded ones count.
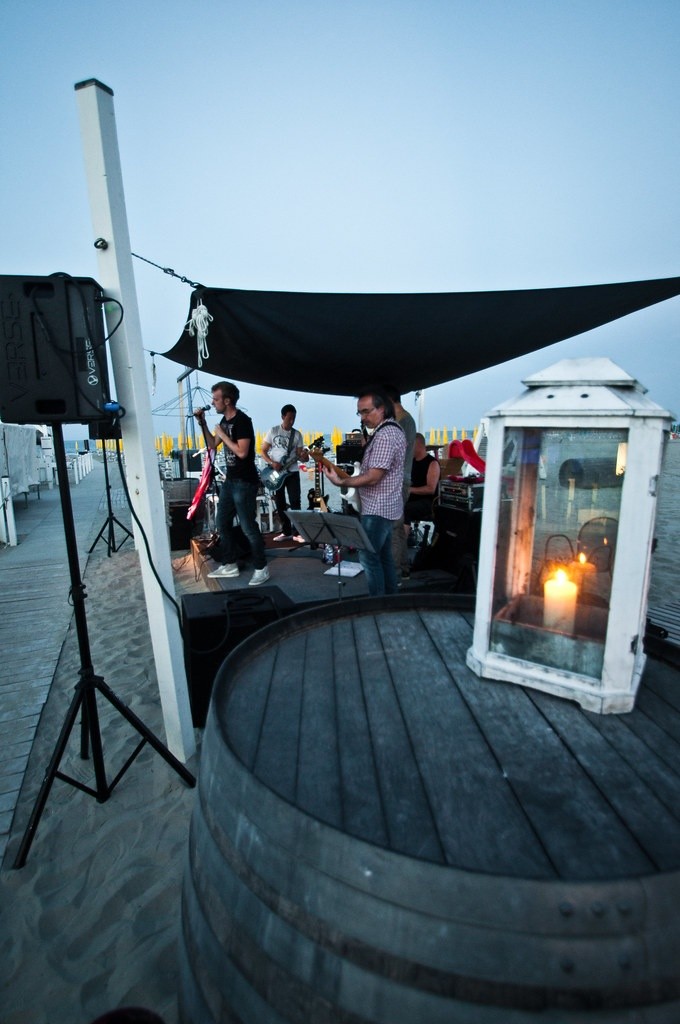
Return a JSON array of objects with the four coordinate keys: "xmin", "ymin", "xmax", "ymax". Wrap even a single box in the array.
[
  {"xmin": 207, "ymin": 563, "xmax": 239, "ymax": 577},
  {"xmin": 248, "ymin": 565, "xmax": 270, "ymax": 586},
  {"xmin": 292, "ymin": 535, "xmax": 306, "ymax": 544},
  {"xmin": 273, "ymin": 533, "xmax": 293, "ymax": 541}
]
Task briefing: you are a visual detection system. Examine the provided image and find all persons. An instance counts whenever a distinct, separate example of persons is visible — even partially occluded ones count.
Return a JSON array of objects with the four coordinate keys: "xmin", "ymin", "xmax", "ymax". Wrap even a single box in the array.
[
  {"xmin": 323, "ymin": 390, "xmax": 407, "ymax": 595},
  {"xmin": 403, "ymin": 432, "xmax": 440, "ymax": 526},
  {"xmin": 262, "ymin": 404, "xmax": 309, "ymax": 543},
  {"xmin": 194, "ymin": 381, "xmax": 271, "ymax": 585},
  {"xmin": 383, "ymin": 389, "xmax": 416, "ymax": 586}
]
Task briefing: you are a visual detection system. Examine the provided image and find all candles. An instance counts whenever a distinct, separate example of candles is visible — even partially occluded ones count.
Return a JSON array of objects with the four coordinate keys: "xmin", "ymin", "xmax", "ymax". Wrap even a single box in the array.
[{"xmin": 541, "ymin": 565, "xmax": 581, "ymax": 634}]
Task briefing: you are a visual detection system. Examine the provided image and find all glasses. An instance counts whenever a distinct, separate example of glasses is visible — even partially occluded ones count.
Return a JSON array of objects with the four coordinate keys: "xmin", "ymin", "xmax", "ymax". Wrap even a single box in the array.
[{"xmin": 356, "ymin": 406, "xmax": 375, "ymax": 418}]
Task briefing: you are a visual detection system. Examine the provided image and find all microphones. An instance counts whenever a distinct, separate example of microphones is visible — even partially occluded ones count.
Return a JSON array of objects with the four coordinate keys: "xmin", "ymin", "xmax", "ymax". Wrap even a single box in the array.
[{"xmin": 188, "ymin": 405, "xmax": 211, "ymax": 418}]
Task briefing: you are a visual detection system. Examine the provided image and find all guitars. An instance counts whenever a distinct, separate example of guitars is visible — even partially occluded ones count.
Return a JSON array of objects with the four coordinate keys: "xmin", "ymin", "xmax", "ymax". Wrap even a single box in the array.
[
  {"xmin": 261, "ymin": 436, "xmax": 325, "ymax": 490},
  {"xmin": 310, "ymin": 445, "xmax": 361, "ymax": 513},
  {"xmin": 307, "ymin": 440, "xmax": 331, "ymax": 513}
]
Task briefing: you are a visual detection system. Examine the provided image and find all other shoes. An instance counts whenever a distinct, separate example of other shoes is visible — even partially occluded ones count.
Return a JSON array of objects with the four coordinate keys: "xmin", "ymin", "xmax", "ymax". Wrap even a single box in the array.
[{"xmin": 400, "ymin": 570, "xmax": 410, "ymax": 580}]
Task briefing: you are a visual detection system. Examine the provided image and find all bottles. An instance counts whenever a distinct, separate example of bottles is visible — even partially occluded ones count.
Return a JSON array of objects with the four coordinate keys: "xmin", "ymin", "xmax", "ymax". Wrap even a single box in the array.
[{"xmin": 323, "ymin": 544, "xmax": 340, "ymax": 565}]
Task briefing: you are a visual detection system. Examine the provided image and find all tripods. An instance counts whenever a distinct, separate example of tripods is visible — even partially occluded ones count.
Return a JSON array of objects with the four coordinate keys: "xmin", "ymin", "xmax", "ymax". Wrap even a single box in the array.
[
  {"xmin": 89, "ymin": 441, "xmax": 134, "ymax": 557},
  {"xmin": 11, "ymin": 423, "xmax": 196, "ymax": 869}
]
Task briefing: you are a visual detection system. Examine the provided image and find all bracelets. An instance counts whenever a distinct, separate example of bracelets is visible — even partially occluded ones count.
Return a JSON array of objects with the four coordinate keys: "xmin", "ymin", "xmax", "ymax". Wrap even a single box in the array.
[{"xmin": 198, "ymin": 420, "xmax": 207, "ymax": 426}]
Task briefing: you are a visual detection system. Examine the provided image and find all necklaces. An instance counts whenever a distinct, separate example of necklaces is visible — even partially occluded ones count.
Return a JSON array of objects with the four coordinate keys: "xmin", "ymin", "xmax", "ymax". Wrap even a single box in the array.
[{"xmin": 228, "ymin": 412, "xmax": 235, "ymax": 420}]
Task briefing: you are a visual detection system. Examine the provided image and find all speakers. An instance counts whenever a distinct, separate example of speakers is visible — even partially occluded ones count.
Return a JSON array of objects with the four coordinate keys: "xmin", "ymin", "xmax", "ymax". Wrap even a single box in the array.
[
  {"xmin": 89, "ymin": 402, "xmax": 123, "ymax": 439},
  {"xmin": 180, "ymin": 584, "xmax": 295, "ymax": 729},
  {"xmin": 0, "ymin": 272, "xmax": 110, "ymax": 425}
]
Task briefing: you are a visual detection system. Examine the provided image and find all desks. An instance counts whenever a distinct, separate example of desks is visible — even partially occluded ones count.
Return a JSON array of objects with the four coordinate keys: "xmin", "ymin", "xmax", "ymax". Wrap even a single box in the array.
[{"xmin": 206, "ymin": 493, "xmax": 275, "ymax": 533}]
